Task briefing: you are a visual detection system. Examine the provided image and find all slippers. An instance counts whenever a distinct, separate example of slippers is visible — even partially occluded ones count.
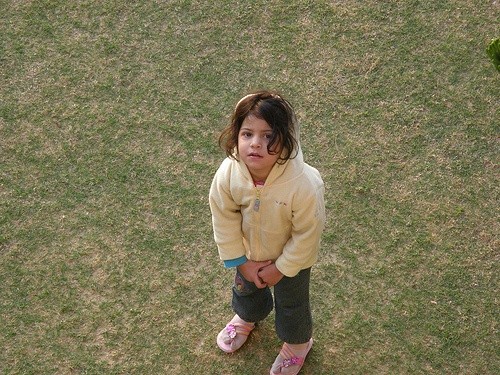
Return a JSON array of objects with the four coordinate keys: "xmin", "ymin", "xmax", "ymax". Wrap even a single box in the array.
[
  {"xmin": 269, "ymin": 337, "xmax": 313, "ymax": 375},
  {"xmin": 217, "ymin": 314, "xmax": 255, "ymax": 352}
]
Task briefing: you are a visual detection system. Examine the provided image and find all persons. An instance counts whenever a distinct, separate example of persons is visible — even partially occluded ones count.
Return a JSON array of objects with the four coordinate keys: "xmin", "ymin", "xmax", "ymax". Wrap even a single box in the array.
[{"xmin": 208, "ymin": 91, "xmax": 326, "ymax": 375}]
